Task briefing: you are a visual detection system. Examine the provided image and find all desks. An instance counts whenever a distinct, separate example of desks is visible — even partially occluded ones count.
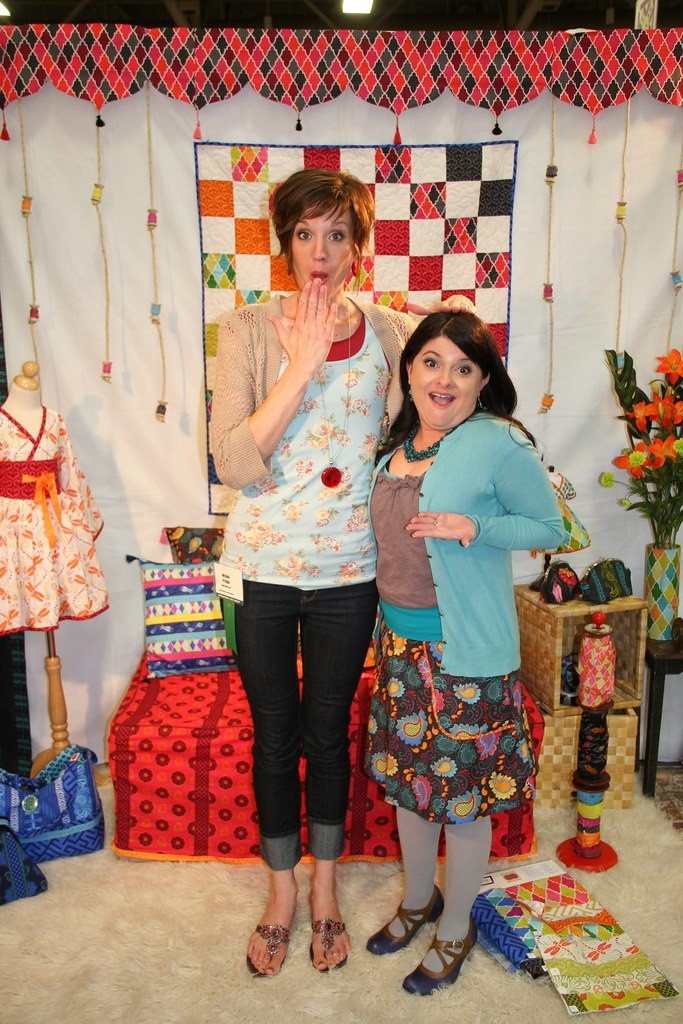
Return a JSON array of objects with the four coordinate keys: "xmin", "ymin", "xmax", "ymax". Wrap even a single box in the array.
[{"xmin": 632, "ymin": 632, "xmax": 683, "ymax": 799}]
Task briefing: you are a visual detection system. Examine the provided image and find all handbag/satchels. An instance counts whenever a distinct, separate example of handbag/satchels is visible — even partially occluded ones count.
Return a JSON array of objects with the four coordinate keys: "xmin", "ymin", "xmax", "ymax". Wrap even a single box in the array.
[
  {"xmin": 576, "ymin": 556, "xmax": 632, "ymax": 604},
  {"xmin": 0, "ymin": 746, "xmax": 104, "ymax": 863},
  {"xmin": 559, "ymin": 656, "xmax": 579, "ymax": 705},
  {"xmin": 0, "ymin": 815, "xmax": 48, "ymax": 904},
  {"xmin": 539, "ymin": 557, "xmax": 581, "ymax": 604}
]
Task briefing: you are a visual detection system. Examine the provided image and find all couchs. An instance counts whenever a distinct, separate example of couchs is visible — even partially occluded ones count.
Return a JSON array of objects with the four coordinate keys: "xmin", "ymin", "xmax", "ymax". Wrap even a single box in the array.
[{"xmin": 108, "ymin": 578, "xmax": 543, "ymax": 865}]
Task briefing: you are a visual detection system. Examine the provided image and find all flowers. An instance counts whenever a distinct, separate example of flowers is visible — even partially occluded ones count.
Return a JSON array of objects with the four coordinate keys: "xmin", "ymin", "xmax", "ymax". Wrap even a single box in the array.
[{"xmin": 600, "ymin": 350, "xmax": 683, "ymax": 550}]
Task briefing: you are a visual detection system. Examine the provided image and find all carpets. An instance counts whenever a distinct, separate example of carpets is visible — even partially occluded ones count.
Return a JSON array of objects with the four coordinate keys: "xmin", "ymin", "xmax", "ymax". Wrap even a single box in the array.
[{"xmin": 0, "ymin": 761, "xmax": 682, "ymax": 1024}]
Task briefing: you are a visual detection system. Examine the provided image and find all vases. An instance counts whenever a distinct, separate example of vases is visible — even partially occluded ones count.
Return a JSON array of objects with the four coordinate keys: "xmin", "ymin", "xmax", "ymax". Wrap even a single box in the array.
[{"xmin": 644, "ymin": 542, "xmax": 680, "ymax": 640}]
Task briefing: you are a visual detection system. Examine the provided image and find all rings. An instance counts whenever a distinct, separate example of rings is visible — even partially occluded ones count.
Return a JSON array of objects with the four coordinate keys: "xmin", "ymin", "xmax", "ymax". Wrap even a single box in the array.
[{"xmin": 433, "ymin": 519, "xmax": 438, "ymax": 525}]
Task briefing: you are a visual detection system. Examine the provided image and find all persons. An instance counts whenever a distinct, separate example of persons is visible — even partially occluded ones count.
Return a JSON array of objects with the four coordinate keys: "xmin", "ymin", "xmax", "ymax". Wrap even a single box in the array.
[
  {"xmin": 365, "ymin": 312, "xmax": 566, "ymax": 992},
  {"xmin": 0, "ymin": 382, "xmax": 109, "ymax": 635},
  {"xmin": 208, "ymin": 169, "xmax": 477, "ymax": 978}
]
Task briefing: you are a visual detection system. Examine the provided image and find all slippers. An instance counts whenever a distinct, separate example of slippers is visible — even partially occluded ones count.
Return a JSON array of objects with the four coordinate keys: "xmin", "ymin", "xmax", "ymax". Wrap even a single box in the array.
[
  {"xmin": 246, "ymin": 924, "xmax": 290, "ymax": 978},
  {"xmin": 310, "ymin": 919, "xmax": 349, "ymax": 973}
]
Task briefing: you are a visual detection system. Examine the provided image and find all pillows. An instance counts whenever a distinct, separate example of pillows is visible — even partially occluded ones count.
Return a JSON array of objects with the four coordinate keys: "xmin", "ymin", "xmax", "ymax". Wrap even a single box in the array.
[{"xmin": 125, "ymin": 528, "xmax": 239, "ymax": 683}]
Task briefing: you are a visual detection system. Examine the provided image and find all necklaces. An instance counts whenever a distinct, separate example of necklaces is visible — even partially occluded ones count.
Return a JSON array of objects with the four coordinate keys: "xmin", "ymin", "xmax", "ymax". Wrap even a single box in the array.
[
  {"xmin": 404, "ymin": 420, "xmax": 457, "ymax": 463},
  {"xmin": 318, "ymin": 299, "xmax": 350, "ymax": 487}
]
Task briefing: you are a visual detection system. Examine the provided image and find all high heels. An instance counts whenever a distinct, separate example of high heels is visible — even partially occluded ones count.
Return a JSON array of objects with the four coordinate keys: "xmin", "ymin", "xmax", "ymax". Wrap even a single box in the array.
[
  {"xmin": 366, "ymin": 884, "xmax": 445, "ymax": 955},
  {"xmin": 401, "ymin": 911, "xmax": 478, "ymax": 997}
]
